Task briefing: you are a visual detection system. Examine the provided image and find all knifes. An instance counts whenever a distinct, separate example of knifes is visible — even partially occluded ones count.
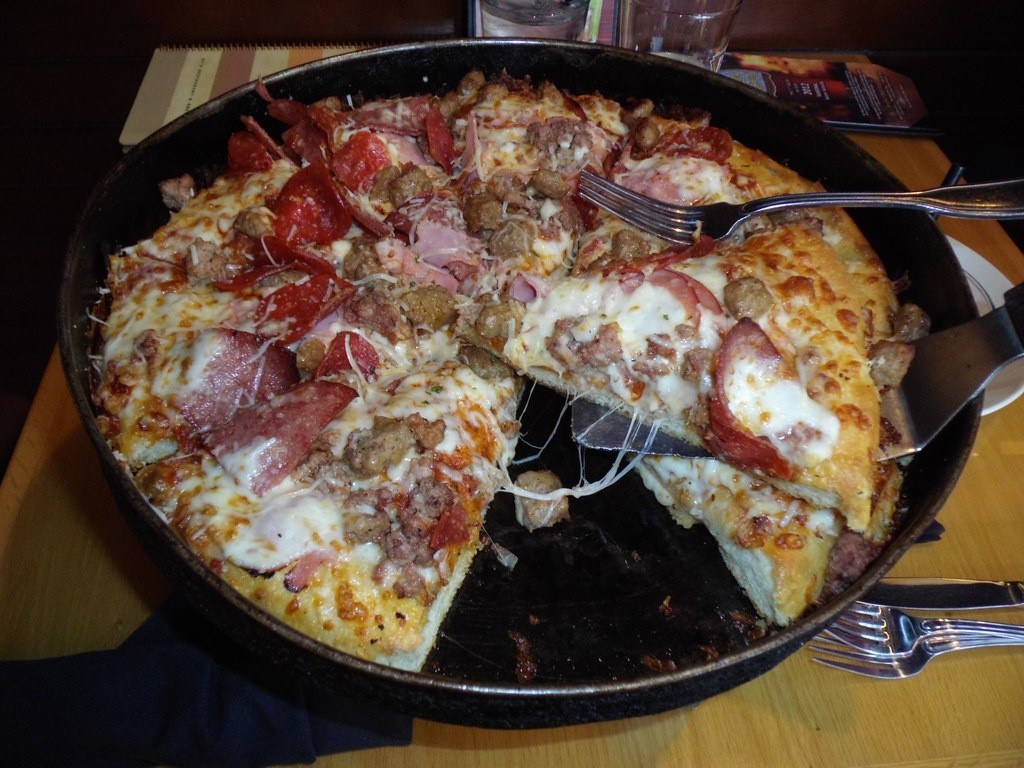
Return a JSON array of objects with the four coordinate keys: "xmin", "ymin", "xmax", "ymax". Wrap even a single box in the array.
[{"xmin": 861, "ymin": 577, "xmax": 1024, "ymax": 611}]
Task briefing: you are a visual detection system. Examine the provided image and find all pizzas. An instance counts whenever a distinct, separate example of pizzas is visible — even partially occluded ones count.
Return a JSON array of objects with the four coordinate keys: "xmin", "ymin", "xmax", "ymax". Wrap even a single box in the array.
[{"xmin": 97, "ymin": 67, "xmax": 929, "ymax": 674}]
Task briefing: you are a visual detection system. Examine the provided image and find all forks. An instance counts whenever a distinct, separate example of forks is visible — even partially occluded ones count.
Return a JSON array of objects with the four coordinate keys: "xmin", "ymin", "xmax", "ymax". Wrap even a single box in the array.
[
  {"xmin": 578, "ymin": 167, "xmax": 1024, "ymax": 245},
  {"xmin": 823, "ymin": 601, "xmax": 1024, "ymax": 658},
  {"xmin": 808, "ymin": 634, "xmax": 1024, "ymax": 680}
]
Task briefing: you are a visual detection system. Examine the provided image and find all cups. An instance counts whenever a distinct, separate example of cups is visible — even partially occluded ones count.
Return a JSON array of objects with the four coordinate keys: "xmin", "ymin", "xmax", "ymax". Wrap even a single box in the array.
[
  {"xmin": 619, "ymin": 0, "xmax": 742, "ymax": 73},
  {"xmin": 480, "ymin": 0, "xmax": 591, "ymax": 45}
]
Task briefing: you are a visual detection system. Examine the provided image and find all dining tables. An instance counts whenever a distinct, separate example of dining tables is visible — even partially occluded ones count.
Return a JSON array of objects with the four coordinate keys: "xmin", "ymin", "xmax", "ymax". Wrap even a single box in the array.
[{"xmin": 0, "ymin": 43, "xmax": 1024, "ymax": 768}]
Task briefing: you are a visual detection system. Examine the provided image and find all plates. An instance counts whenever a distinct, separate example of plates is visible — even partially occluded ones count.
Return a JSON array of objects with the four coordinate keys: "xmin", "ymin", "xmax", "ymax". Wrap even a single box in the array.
[
  {"xmin": 945, "ymin": 235, "xmax": 1024, "ymax": 416},
  {"xmin": 59, "ymin": 36, "xmax": 986, "ymax": 729}
]
